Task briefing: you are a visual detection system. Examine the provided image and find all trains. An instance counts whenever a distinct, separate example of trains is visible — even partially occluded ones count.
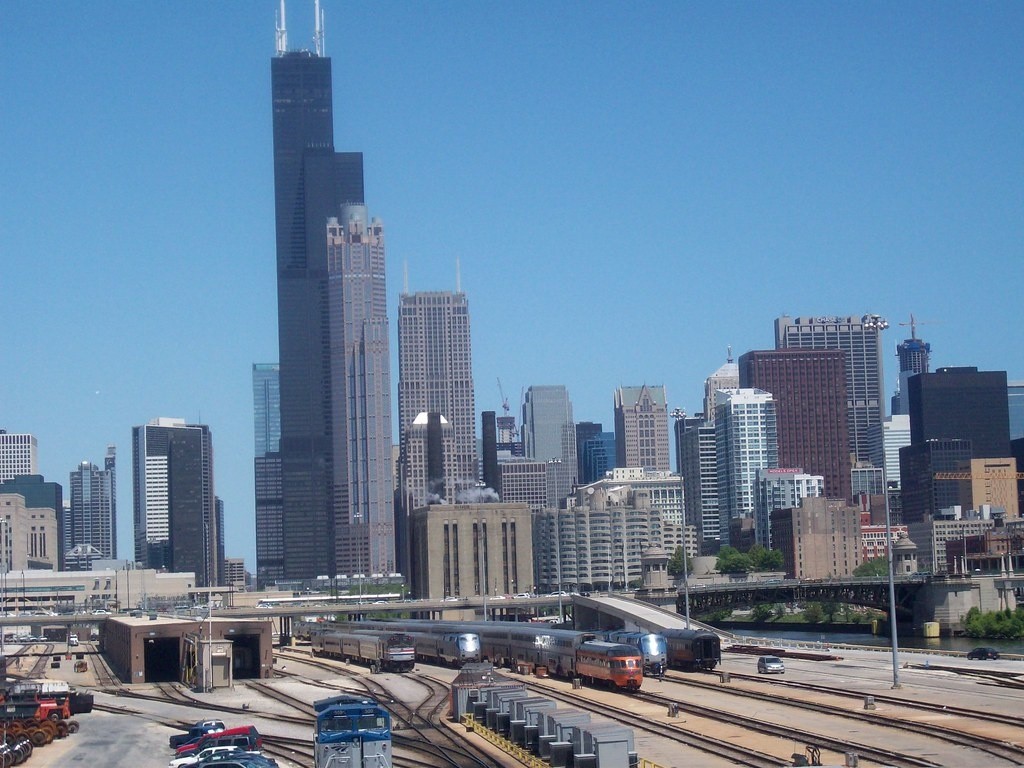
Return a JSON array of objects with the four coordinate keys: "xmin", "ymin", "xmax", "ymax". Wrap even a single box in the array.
[
  {"xmin": 310, "ymin": 626, "xmax": 416, "ymax": 674},
  {"xmin": 436, "ymin": 619, "xmax": 670, "ymax": 680},
  {"xmin": 312, "ymin": 694, "xmax": 392, "ymax": 768},
  {"xmin": 343, "ymin": 617, "xmax": 645, "ymax": 694},
  {"xmin": 349, "ymin": 629, "xmax": 482, "ymax": 670}
]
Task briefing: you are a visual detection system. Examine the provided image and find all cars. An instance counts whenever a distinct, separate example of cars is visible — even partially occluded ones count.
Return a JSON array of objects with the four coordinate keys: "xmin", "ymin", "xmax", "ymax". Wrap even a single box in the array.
[
  {"xmin": 967, "ymin": 646, "xmax": 1001, "ymax": 661},
  {"xmin": 1, "ymin": 604, "xmax": 209, "ymax": 617},
  {"xmin": 373, "ymin": 600, "xmax": 389, "ymax": 605},
  {"xmin": 488, "ymin": 595, "xmax": 506, "ymax": 601},
  {"xmin": 440, "ymin": 596, "xmax": 458, "ymax": 602},
  {"xmin": 70, "ymin": 634, "xmax": 79, "ymax": 646},
  {"xmin": 757, "ymin": 656, "xmax": 785, "ymax": 676},
  {"xmin": 4, "ymin": 632, "xmax": 48, "ymax": 643},
  {"xmin": 504, "ymin": 588, "xmax": 591, "ymax": 602},
  {"xmin": 256, "ymin": 602, "xmax": 274, "ymax": 609},
  {"xmin": 169, "ymin": 718, "xmax": 279, "ymax": 768}
]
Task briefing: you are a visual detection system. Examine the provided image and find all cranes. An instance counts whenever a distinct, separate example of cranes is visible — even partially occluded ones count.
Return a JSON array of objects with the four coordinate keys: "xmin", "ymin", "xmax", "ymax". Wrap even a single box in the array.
[
  {"xmin": 900, "ymin": 313, "xmax": 943, "ymax": 340},
  {"xmin": 495, "ymin": 376, "xmax": 510, "ymax": 417}
]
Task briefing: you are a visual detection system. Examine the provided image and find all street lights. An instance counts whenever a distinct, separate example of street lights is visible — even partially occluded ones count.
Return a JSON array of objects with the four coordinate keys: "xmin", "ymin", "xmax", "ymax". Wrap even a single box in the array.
[
  {"xmin": 353, "ymin": 512, "xmax": 362, "ymax": 606},
  {"xmin": 401, "ymin": 584, "xmax": 405, "ymax": 603},
  {"xmin": 859, "ymin": 311, "xmax": 905, "ymax": 691},
  {"xmin": 669, "ymin": 407, "xmax": 687, "ymax": 631},
  {"xmin": 511, "ymin": 580, "xmax": 514, "ymax": 595}
]
